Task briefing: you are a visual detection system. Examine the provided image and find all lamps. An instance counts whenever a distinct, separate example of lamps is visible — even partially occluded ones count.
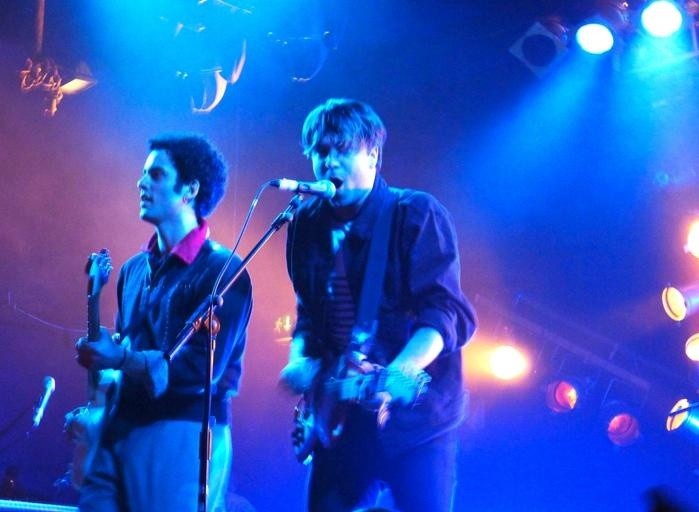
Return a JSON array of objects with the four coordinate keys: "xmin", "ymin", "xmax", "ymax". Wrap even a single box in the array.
[{"xmin": 541, "ymin": 269, "xmax": 699, "ymax": 450}]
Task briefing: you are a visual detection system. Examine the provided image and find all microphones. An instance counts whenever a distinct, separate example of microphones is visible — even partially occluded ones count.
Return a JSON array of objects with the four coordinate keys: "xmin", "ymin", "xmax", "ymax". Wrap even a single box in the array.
[
  {"xmin": 271, "ymin": 178, "xmax": 337, "ymax": 200},
  {"xmin": 32, "ymin": 375, "xmax": 55, "ymax": 426}
]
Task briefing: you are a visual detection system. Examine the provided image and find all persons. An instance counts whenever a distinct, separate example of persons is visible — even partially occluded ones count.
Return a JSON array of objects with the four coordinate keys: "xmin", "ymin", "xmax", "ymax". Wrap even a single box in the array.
[
  {"xmin": 279, "ymin": 99, "xmax": 478, "ymax": 511},
  {"xmin": 63, "ymin": 134, "xmax": 253, "ymax": 512}
]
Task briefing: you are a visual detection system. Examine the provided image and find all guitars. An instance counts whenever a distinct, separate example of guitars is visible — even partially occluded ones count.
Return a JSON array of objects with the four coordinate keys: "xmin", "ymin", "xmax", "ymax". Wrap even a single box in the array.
[
  {"xmin": 291, "ymin": 346, "xmax": 433, "ymax": 465},
  {"xmin": 63, "ymin": 247, "xmax": 114, "ymax": 492}
]
